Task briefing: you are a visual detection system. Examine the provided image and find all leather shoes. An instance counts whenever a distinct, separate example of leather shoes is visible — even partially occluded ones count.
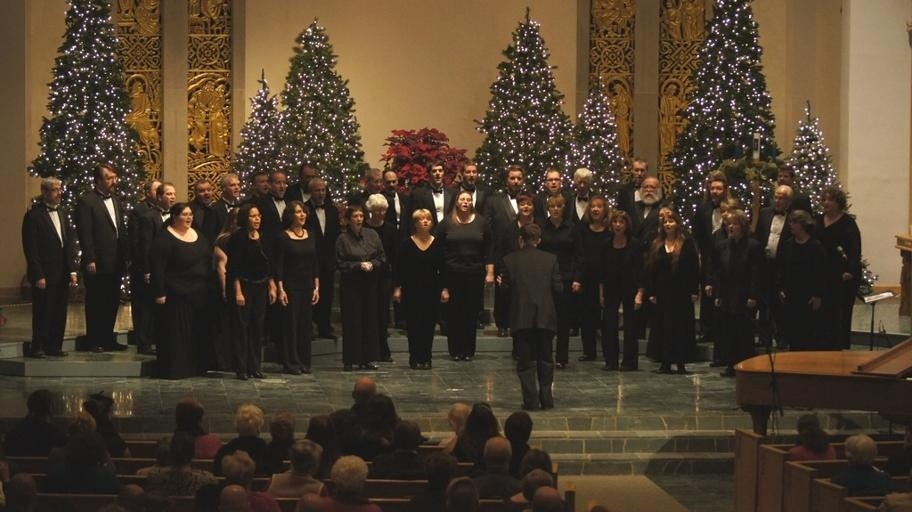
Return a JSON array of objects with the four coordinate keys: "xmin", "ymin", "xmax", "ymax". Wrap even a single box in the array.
[
  {"xmin": 105, "ymin": 341, "xmax": 128, "ymax": 351},
  {"xmin": 520, "ymin": 404, "xmax": 539, "ymax": 411},
  {"xmin": 709, "ymin": 361, "xmax": 725, "ymax": 367},
  {"xmin": 498, "ymin": 327, "xmax": 508, "ymax": 337},
  {"xmin": 476, "ymin": 322, "xmax": 485, "ymax": 328},
  {"xmin": 301, "ymin": 367, "xmax": 312, "ymax": 373},
  {"xmin": 32, "ymin": 350, "xmax": 46, "ymax": 358},
  {"xmin": 541, "ymin": 404, "xmax": 554, "ymax": 411},
  {"xmin": 87, "ymin": 345, "xmax": 103, "ymax": 352},
  {"xmin": 137, "ymin": 346, "xmax": 157, "ymax": 355},
  {"xmin": 423, "ymin": 362, "xmax": 432, "ymax": 369},
  {"xmin": 555, "ymin": 362, "xmax": 562, "ymax": 368},
  {"xmin": 578, "ymin": 355, "xmax": 590, "ymax": 361},
  {"xmin": 284, "ymin": 368, "xmax": 302, "ymax": 375},
  {"xmin": 453, "ymin": 356, "xmax": 460, "ymax": 361},
  {"xmin": 465, "ymin": 357, "xmax": 472, "ymax": 361},
  {"xmin": 411, "ymin": 363, "xmax": 423, "ymax": 369},
  {"xmin": 45, "ymin": 351, "xmax": 69, "ymax": 357}
]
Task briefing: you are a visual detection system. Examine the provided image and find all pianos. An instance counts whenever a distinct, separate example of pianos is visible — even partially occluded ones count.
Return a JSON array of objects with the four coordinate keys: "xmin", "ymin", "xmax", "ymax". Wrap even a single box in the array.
[{"xmin": 734, "ymin": 339, "xmax": 906, "ymax": 435}]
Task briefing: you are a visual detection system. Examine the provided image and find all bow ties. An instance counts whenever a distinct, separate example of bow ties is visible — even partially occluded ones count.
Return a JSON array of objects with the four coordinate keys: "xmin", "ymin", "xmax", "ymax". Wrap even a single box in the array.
[
  {"xmin": 431, "ymin": 187, "xmax": 443, "ymax": 194},
  {"xmin": 100, "ymin": 191, "xmax": 112, "ymax": 201},
  {"xmin": 508, "ymin": 193, "xmax": 516, "ymax": 199},
  {"xmin": 463, "ymin": 187, "xmax": 475, "ymax": 193},
  {"xmin": 45, "ymin": 204, "xmax": 60, "ymax": 213},
  {"xmin": 160, "ymin": 210, "xmax": 171, "ymax": 216},
  {"xmin": 225, "ymin": 200, "xmax": 234, "ymax": 209},
  {"xmin": 577, "ymin": 197, "xmax": 588, "ymax": 202},
  {"xmin": 275, "ymin": 196, "xmax": 284, "ymax": 201},
  {"xmin": 315, "ymin": 204, "xmax": 325, "ymax": 209},
  {"xmin": 388, "ymin": 191, "xmax": 395, "ymax": 197}
]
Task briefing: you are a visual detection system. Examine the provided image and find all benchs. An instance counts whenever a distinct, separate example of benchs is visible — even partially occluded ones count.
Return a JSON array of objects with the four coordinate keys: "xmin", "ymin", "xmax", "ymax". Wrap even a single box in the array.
[{"xmin": 728, "ymin": 428, "xmax": 912, "ymax": 512}]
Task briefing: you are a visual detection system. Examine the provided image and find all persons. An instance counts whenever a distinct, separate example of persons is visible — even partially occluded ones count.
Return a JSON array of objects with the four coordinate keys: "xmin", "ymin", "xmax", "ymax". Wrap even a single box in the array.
[
  {"xmin": 790, "ymin": 411, "xmax": 911, "ymax": 496},
  {"xmin": 123, "ymin": 77, "xmax": 162, "ymax": 152},
  {"xmin": 1, "ymin": 378, "xmax": 576, "ymax": 512},
  {"xmin": 657, "ymin": 79, "xmax": 688, "ymax": 158},
  {"xmin": 23, "ymin": 160, "xmax": 861, "ymax": 410},
  {"xmin": 609, "ymin": 79, "xmax": 633, "ymax": 156},
  {"xmin": 187, "ymin": 80, "xmax": 215, "ymax": 155},
  {"xmin": 208, "ymin": 83, "xmax": 230, "ymax": 158}
]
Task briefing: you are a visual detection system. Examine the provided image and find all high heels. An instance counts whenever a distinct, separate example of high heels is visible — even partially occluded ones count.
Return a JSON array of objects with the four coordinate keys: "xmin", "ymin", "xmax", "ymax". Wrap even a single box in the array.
[
  {"xmin": 656, "ymin": 361, "xmax": 671, "ymax": 373},
  {"xmin": 358, "ymin": 360, "xmax": 379, "ymax": 369},
  {"xmin": 249, "ymin": 371, "xmax": 263, "ymax": 378},
  {"xmin": 344, "ymin": 363, "xmax": 352, "ymax": 371},
  {"xmin": 677, "ymin": 362, "xmax": 688, "ymax": 374},
  {"xmin": 602, "ymin": 361, "xmax": 619, "ymax": 371},
  {"xmin": 720, "ymin": 368, "xmax": 735, "ymax": 377},
  {"xmin": 236, "ymin": 372, "xmax": 249, "ymax": 380}
]
